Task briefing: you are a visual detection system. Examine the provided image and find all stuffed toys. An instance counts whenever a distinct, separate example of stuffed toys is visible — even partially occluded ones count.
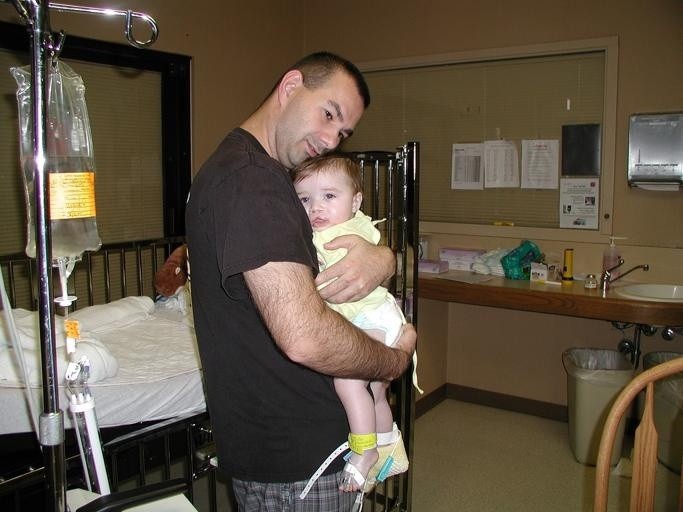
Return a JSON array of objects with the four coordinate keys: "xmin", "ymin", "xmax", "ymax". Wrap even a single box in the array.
[{"xmin": 152, "ymin": 242, "xmax": 189, "ymax": 298}]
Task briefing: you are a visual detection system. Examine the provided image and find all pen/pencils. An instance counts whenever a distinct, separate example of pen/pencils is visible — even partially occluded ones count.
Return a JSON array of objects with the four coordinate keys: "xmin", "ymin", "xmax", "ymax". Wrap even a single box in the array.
[{"xmin": 537, "ymin": 279, "xmax": 562, "ymax": 286}]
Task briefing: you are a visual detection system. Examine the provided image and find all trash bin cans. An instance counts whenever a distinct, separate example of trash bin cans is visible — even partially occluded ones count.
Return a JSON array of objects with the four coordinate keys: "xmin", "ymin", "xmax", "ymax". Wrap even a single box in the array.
[
  {"xmin": 562, "ymin": 348, "xmax": 635, "ymax": 466},
  {"xmin": 641, "ymin": 351, "xmax": 683, "ymax": 475}
]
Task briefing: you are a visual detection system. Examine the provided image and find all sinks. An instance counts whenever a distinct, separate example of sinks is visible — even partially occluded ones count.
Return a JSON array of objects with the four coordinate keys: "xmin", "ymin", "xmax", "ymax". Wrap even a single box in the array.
[{"xmin": 614, "ymin": 280, "xmax": 683, "ymax": 303}]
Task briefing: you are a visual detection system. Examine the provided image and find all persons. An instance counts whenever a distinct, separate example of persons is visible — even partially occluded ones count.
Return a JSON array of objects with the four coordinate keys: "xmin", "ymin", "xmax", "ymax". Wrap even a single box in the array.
[
  {"xmin": 288, "ymin": 153, "xmax": 411, "ymax": 495},
  {"xmin": 183, "ymin": 51, "xmax": 418, "ymax": 512}
]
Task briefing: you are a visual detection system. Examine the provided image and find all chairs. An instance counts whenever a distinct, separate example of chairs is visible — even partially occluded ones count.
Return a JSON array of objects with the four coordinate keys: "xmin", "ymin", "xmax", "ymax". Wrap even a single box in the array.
[{"xmin": 587, "ymin": 349, "xmax": 683, "ymax": 510}]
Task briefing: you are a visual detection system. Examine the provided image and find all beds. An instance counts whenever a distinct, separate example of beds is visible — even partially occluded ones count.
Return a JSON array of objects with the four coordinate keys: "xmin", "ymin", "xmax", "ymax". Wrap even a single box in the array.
[{"xmin": 1, "ymin": 236, "xmax": 208, "ymax": 511}]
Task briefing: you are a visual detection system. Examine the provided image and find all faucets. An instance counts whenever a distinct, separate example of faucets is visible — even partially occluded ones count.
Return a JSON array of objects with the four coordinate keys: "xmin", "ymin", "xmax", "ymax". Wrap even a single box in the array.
[{"xmin": 600, "ymin": 258, "xmax": 649, "ymax": 290}]
